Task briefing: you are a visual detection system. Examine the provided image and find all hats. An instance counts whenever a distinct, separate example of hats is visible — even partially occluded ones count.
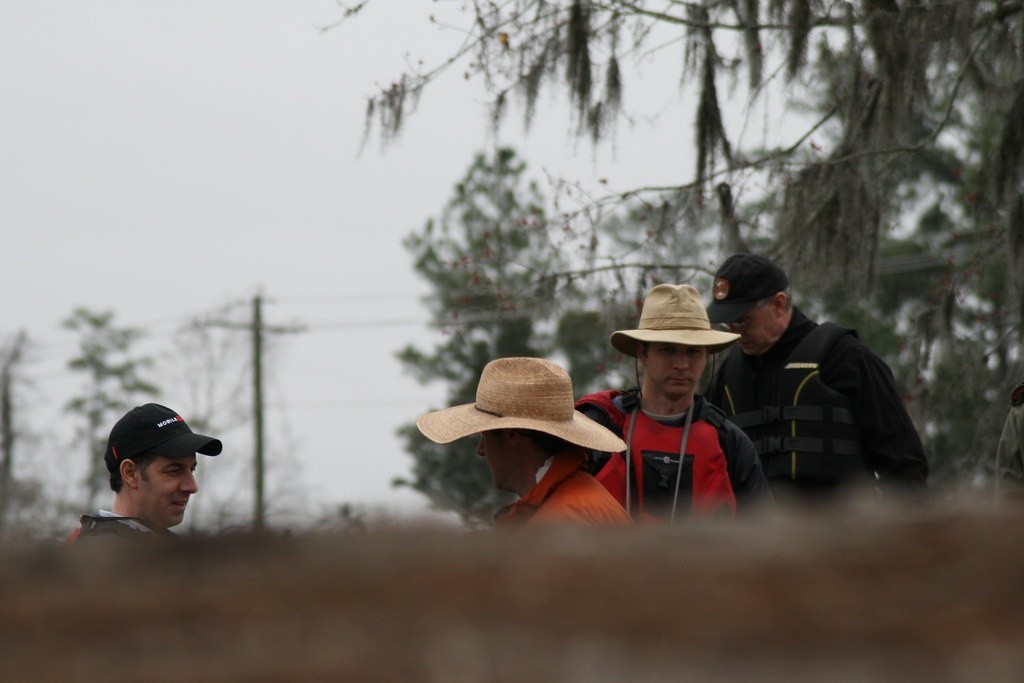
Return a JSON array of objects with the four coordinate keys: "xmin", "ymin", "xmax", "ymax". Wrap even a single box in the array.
[
  {"xmin": 610, "ymin": 283, "xmax": 742, "ymax": 357},
  {"xmin": 417, "ymin": 356, "xmax": 627, "ymax": 452},
  {"xmin": 707, "ymin": 252, "xmax": 786, "ymax": 323},
  {"xmin": 105, "ymin": 404, "xmax": 224, "ymax": 473}
]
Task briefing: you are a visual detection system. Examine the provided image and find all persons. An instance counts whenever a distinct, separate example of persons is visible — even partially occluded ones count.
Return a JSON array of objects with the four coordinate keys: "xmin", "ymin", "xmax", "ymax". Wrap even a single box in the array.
[
  {"xmin": 415, "ymin": 356, "xmax": 640, "ymax": 534},
  {"xmin": 994, "ymin": 380, "xmax": 1024, "ymax": 512},
  {"xmin": 74, "ymin": 403, "xmax": 223, "ymax": 546},
  {"xmin": 572, "ymin": 284, "xmax": 776, "ymax": 532},
  {"xmin": 707, "ymin": 254, "xmax": 931, "ymax": 517}
]
